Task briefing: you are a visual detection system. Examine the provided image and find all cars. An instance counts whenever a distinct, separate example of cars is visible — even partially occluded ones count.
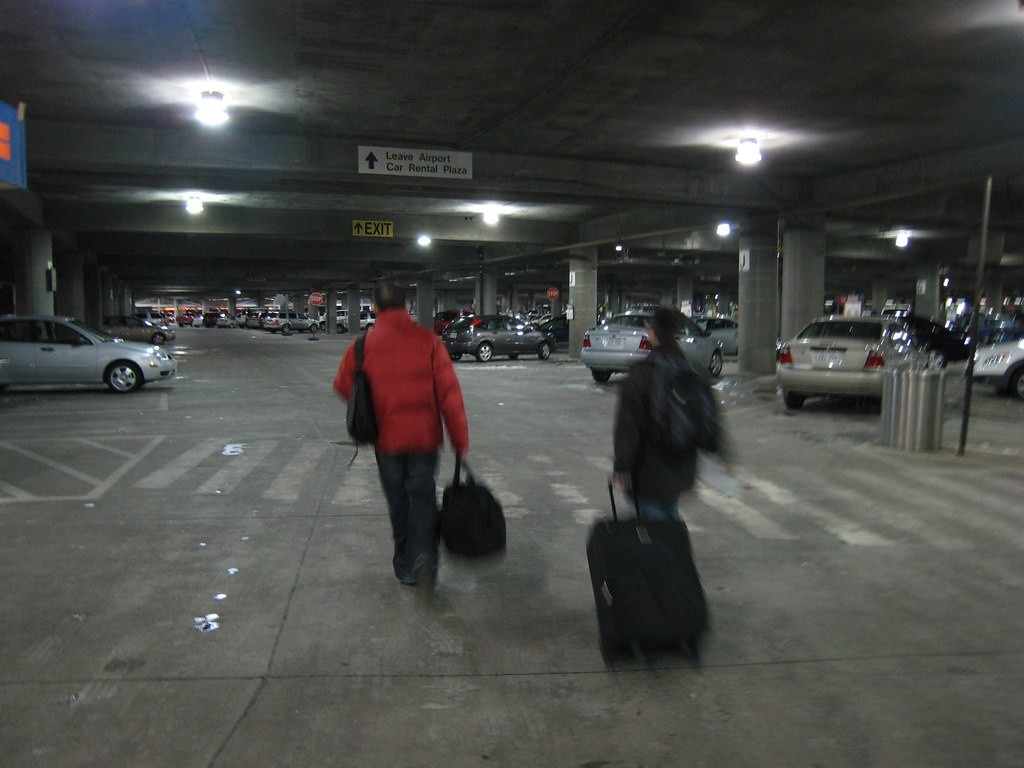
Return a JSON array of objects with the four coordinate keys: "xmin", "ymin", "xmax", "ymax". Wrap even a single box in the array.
[
  {"xmin": 436, "ymin": 308, "xmax": 478, "ymax": 337},
  {"xmin": 579, "ymin": 306, "xmax": 723, "ymax": 381},
  {"xmin": 137, "ymin": 309, "xmax": 262, "ymax": 329},
  {"xmin": 692, "ymin": 316, "xmax": 741, "ymax": 357},
  {"xmin": 1, "ymin": 315, "xmax": 178, "ymax": 393},
  {"xmin": 319, "ymin": 310, "xmax": 376, "ymax": 331},
  {"xmin": 779, "ymin": 303, "xmax": 1024, "ymax": 413},
  {"xmin": 97, "ymin": 315, "xmax": 176, "ymax": 344},
  {"xmin": 967, "ymin": 336, "xmax": 1024, "ymax": 402},
  {"xmin": 499, "ymin": 309, "xmax": 607, "ymax": 344},
  {"xmin": 442, "ymin": 314, "xmax": 556, "ymax": 363}
]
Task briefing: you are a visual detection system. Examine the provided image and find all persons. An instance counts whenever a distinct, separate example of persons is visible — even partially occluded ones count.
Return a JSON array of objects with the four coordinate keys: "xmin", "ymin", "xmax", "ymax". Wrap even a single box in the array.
[
  {"xmin": 613, "ymin": 308, "xmax": 719, "ymax": 522},
  {"xmin": 334, "ymin": 280, "xmax": 469, "ymax": 587}
]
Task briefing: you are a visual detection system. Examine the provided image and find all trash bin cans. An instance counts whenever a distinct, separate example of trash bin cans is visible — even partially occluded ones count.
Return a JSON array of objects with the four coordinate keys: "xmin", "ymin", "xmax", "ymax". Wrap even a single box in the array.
[{"xmin": 878, "ymin": 364, "xmax": 948, "ymax": 454}]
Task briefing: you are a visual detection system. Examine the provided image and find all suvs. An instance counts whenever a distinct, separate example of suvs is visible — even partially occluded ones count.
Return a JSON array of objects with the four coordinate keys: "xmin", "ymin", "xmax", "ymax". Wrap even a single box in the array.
[{"xmin": 263, "ymin": 312, "xmax": 320, "ymax": 334}]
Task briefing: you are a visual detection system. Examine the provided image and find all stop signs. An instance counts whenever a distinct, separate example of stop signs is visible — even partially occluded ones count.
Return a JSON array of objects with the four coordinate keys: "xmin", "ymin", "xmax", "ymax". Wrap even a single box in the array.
[
  {"xmin": 310, "ymin": 292, "xmax": 323, "ymax": 306},
  {"xmin": 547, "ymin": 287, "xmax": 558, "ymax": 301}
]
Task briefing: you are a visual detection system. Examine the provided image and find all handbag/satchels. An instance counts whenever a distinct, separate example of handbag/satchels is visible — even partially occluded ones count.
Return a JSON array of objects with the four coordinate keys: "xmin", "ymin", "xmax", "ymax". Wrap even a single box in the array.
[
  {"xmin": 438, "ymin": 453, "xmax": 506, "ymax": 563},
  {"xmin": 347, "ymin": 331, "xmax": 382, "ymax": 446},
  {"xmin": 656, "ymin": 339, "xmax": 721, "ymax": 452}
]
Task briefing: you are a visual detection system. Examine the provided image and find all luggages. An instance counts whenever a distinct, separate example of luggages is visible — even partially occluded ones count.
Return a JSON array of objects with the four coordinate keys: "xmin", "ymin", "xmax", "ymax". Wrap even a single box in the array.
[{"xmin": 585, "ymin": 479, "xmax": 707, "ymax": 664}]
